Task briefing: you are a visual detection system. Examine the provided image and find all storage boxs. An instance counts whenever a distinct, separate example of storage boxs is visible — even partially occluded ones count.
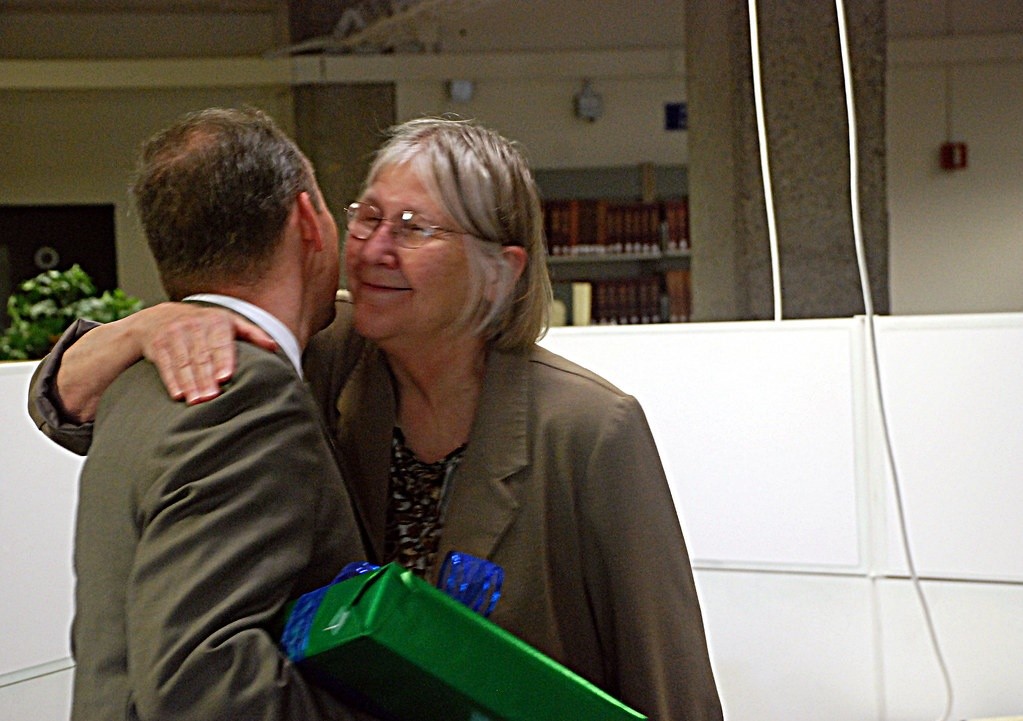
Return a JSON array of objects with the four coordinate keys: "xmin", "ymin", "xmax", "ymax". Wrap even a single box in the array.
[{"xmin": 276, "ymin": 560, "xmax": 645, "ymax": 721}]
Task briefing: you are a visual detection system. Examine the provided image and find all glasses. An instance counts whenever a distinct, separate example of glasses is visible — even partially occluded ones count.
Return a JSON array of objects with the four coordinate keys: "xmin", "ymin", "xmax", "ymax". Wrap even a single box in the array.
[{"xmin": 340, "ymin": 200, "xmax": 509, "ymax": 249}]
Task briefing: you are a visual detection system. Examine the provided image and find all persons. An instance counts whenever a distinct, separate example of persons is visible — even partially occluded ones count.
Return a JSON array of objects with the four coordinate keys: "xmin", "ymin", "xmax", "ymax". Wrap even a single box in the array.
[
  {"xmin": 70, "ymin": 106, "xmax": 479, "ymax": 721},
  {"xmin": 28, "ymin": 118, "xmax": 723, "ymax": 721}
]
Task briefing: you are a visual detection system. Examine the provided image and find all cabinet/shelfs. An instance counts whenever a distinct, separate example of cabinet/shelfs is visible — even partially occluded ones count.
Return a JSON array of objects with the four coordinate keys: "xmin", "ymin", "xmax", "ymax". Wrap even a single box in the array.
[{"xmin": 529, "ymin": 160, "xmax": 699, "ymax": 324}]
